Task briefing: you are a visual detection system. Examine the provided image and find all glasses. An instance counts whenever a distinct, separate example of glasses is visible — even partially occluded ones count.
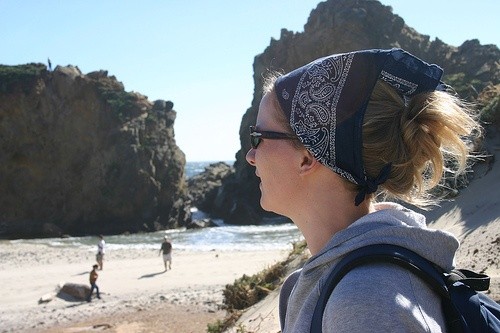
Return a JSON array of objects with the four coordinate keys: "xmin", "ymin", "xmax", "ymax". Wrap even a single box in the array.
[{"xmin": 249, "ymin": 125, "xmax": 298, "ymax": 149}]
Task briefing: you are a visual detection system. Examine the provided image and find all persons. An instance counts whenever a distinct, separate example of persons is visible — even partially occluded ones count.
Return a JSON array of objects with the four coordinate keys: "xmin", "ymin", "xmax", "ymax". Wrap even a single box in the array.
[
  {"xmin": 96, "ymin": 234, "xmax": 105, "ymax": 270},
  {"xmin": 89, "ymin": 265, "xmax": 101, "ymax": 300},
  {"xmin": 246, "ymin": 48, "xmax": 500, "ymax": 333},
  {"xmin": 159, "ymin": 237, "xmax": 173, "ymax": 270}
]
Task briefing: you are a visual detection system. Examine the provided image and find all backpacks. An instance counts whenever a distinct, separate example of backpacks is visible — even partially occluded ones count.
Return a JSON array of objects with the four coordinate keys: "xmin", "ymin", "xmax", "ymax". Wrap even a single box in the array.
[{"xmin": 311, "ymin": 244, "xmax": 500, "ymax": 333}]
